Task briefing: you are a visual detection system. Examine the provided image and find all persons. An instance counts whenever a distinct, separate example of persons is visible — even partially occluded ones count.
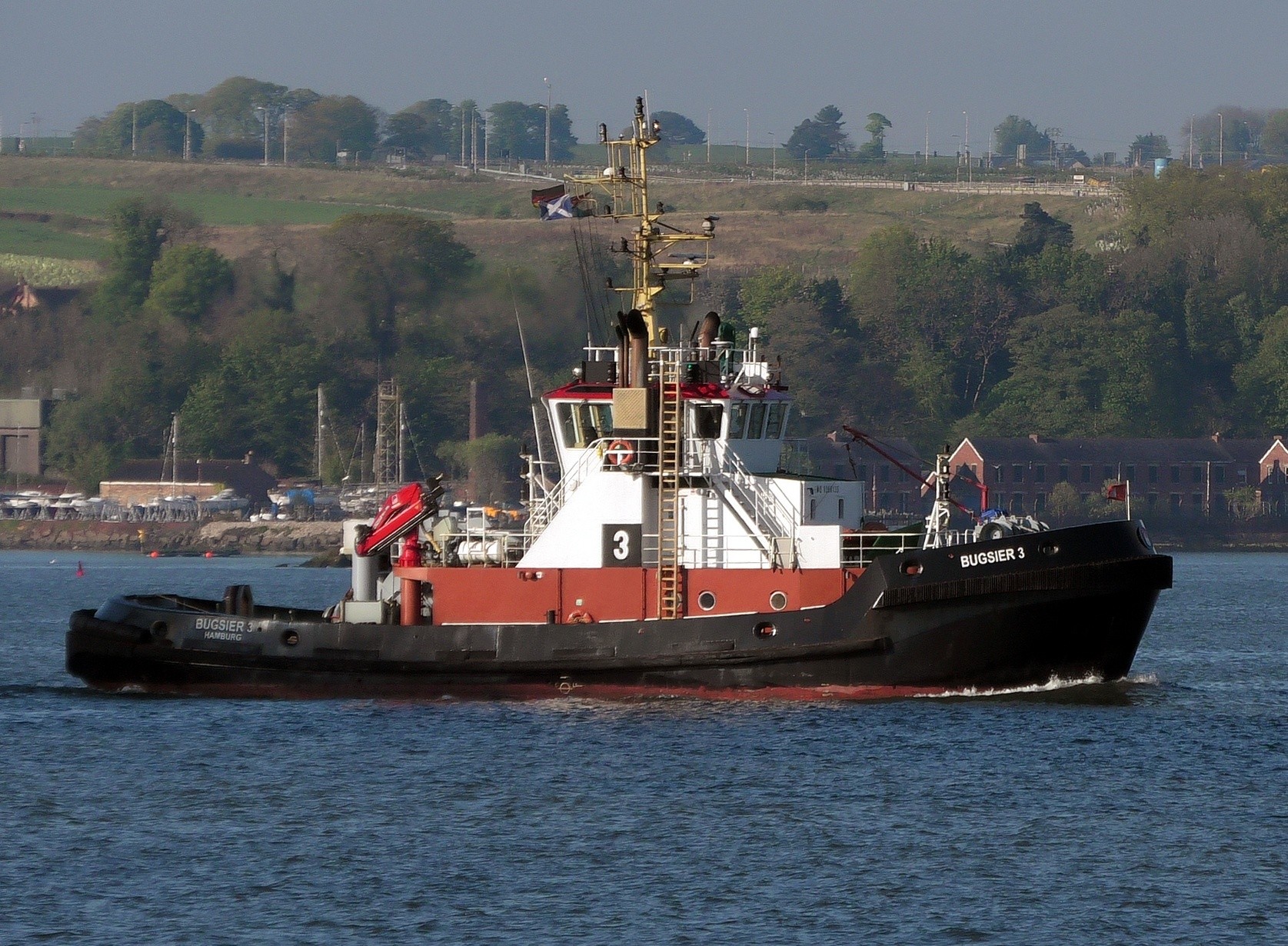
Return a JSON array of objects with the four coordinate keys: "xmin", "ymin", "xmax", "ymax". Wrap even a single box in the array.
[
  {"xmin": 271, "ymin": 503, "xmax": 278, "ymax": 522},
  {"xmin": 254, "ymin": 500, "xmax": 259, "ymax": 513}
]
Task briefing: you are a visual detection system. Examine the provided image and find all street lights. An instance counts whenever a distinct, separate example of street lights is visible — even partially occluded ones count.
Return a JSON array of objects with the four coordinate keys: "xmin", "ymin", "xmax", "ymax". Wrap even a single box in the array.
[
  {"xmin": 768, "ymin": 132, "xmax": 775, "ymax": 182},
  {"xmin": 257, "ymin": 107, "xmax": 269, "ymax": 164},
  {"xmin": 284, "ymin": 104, "xmax": 290, "ymax": 165},
  {"xmin": 805, "ymin": 149, "xmax": 811, "ymax": 185},
  {"xmin": 744, "ymin": 108, "xmax": 749, "ymax": 165},
  {"xmin": 963, "ymin": 111, "xmax": 967, "ymax": 156},
  {"xmin": 707, "ymin": 108, "xmax": 712, "ymax": 162},
  {"xmin": 1217, "ymin": 112, "xmax": 1223, "ymax": 168},
  {"xmin": 197, "ymin": 459, "xmax": 201, "ymax": 498},
  {"xmin": 185, "ymin": 109, "xmax": 197, "ymax": 159},
  {"xmin": 1190, "ymin": 114, "xmax": 1194, "ymax": 172},
  {"xmin": 538, "ymin": 77, "xmax": 550, "ymax": 164},
  {"xmin": 132, "ymin": 98, "xmax": 145, "ymax": 154},
  {"xmin": 926, "ymin": 111, "xmax": 929, "ymax": 164},
  {"xmin": 451, "ymin": 104, "xmax": 491, "ymax": 174}
]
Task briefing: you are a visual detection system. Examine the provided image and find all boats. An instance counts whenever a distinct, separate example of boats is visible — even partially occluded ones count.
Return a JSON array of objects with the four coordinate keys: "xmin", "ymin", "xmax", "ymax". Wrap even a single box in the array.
[
  {"xmin": 354, "ymin": 473, "xmax": 443, "ymax": 554},
  {"xmin": 64, "ymin": 87, "xmax": 1175, "ymax": 704}
]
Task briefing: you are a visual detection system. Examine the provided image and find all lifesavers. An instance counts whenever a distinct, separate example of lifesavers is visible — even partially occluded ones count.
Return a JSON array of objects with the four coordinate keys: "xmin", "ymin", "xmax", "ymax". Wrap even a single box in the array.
[{"xmin": 608, "ymin": 439, "xmax": 634, "ymax": 466}]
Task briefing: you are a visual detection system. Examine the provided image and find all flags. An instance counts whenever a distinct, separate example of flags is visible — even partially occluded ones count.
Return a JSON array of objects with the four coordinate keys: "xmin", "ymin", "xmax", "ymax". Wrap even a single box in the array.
[{"xmin": 1107, "ymin": 484, "xmax": 1127, "ymax": 500}]
